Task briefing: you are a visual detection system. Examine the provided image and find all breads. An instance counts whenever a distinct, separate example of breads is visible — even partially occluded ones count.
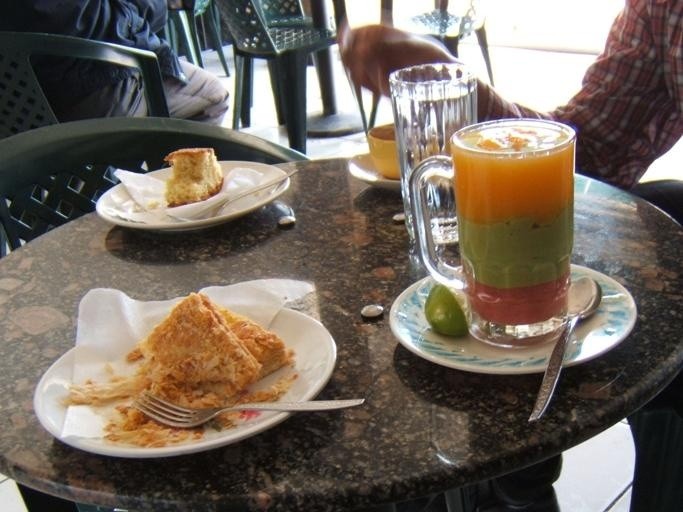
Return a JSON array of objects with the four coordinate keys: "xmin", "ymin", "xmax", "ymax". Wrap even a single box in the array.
[
  {"xmin": 142, "ymin": 293, "xmax": 289, "ymax": 395},
  {"xmin": 162, "ymin": 146, "xmax": 225, "ymax": 208}
]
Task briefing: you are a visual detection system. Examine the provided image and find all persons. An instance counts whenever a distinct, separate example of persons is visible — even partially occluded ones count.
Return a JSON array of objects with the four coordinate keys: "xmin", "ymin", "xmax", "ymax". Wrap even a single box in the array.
[
  {"xmin": 341, "ymin": 0, "xmax": 683, "ymax": 512},
  {"xmin": 0, "ymin": 0, "xmax": 230, "ymax": 126}
]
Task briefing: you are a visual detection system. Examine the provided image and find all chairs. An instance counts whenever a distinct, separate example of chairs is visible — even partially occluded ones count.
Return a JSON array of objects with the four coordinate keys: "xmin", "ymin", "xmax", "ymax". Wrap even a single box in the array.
[
  {"xmin": 0, "ymin": 116, "xmax": 311, "ymax": 259},
  {"xmin": 0, "ymin": 31, "xmax": 170, "ymax": 141},
  {"xmin": 407, "ymin": 0, "xmax": 495, "ymax": 88},
  {"xmin": 163, "ymin": 0, "xmax": 231, "ymax": 77},
  {"xmin": 212, "ymin": 0, "xmax": 341, "ymax": 155}
]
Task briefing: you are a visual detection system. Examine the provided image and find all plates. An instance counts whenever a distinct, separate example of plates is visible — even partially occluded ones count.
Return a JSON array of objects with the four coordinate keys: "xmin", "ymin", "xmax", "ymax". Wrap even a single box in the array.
[
  {"xmin": 96, "ymin": 159, "xmax": 292, "ymax": 233},
  {"xmin": 31, "ymin": 306, "xmax": 339, "ymax": 459},
  {"xmin": 387, "ymin": 262, "xmax": 639, "ymax": 376},
  {"xmin": 349, "ymin": 151, "xmax": 401, "ymax": 191}
]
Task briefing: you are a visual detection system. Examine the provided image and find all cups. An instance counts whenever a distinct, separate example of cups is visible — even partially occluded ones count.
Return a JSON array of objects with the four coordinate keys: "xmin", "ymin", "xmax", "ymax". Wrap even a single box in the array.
[
  {"xmin": 407, "ymin": 117, "xmax": 577, "ymax": 349},
  {"xmin": 368, "ymin": 124, "xmax": 439, "ymax": 180},
  {"xmin": 388, "ymin": 62, "xmax": 479, "ymax": 266}
]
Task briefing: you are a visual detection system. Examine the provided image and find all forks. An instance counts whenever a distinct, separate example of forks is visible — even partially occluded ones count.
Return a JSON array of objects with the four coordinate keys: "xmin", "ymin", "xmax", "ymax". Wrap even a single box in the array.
[
  {"xmin": 134, "ymin": 391, "xmax": 366, "ymax": 429},
  {"xmin": 167, "ymin": 169, "xmax": 300, "ymax": 222}
]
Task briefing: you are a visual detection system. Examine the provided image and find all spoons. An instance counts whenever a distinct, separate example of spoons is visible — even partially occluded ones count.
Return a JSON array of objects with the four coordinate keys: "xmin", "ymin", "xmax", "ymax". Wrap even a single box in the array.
[{"xmin": 527, "ymin": 276, "xmax": 601, "ymax": 421}]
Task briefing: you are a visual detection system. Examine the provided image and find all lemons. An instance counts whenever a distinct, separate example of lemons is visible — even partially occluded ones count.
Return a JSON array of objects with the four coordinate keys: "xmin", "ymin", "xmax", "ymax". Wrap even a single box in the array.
[{"xmin": 424, "ymin": 282, "xmax": 469, "ymax": 339}]
